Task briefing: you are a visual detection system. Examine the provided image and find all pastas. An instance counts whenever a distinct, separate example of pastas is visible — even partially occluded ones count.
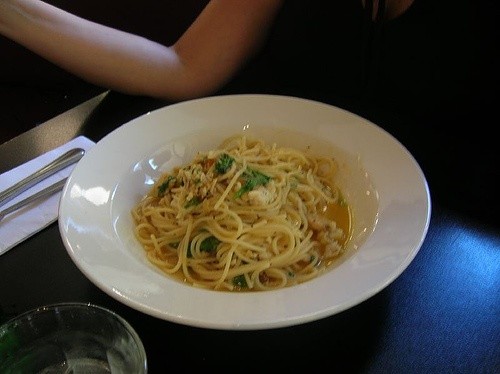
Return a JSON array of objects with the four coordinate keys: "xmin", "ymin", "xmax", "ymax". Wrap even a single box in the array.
[{"xmin": 130, "ymin": 132, "xmax": 344, "ymax": 291}]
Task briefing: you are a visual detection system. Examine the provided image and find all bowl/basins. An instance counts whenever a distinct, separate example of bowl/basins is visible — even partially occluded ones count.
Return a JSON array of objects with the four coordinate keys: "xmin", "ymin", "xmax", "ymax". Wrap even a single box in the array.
[{"xmin": 0, "ymin": 303, "xmax": 148, "ymax": 374}]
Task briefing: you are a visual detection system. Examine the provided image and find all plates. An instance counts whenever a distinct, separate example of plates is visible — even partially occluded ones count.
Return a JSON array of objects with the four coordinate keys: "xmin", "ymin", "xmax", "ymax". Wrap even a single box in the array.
[{"xmin": 58, "ymin": 95, "xmax": 431, "ymax": 331}]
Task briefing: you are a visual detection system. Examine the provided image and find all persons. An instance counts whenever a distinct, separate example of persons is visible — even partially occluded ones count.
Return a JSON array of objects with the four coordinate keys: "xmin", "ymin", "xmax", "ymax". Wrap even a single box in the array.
[{"xmin": 0, "ymin": 1, "xmax": 282, "ymax": 100}]
